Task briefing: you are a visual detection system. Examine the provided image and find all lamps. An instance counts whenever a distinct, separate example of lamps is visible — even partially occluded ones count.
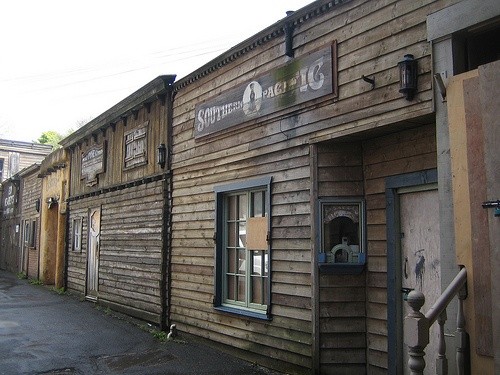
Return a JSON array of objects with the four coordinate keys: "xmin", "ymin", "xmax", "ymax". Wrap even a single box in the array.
[
  {"xmin": 35, "ymin": 199, "xmax": 40, "ymax": 213},
  {"xmin": 396, "ymin": 52, "xmax": 418, "ymax": 101},
  {"xmin": 154, "ymin": 144, "xmax": 167, "ymax": 170}
]
